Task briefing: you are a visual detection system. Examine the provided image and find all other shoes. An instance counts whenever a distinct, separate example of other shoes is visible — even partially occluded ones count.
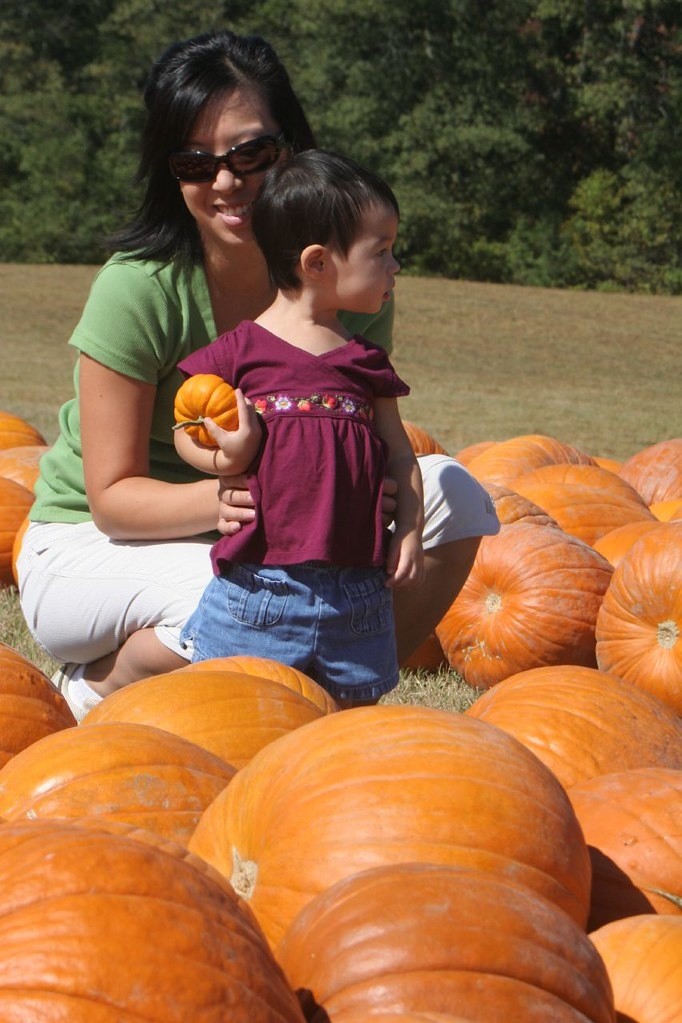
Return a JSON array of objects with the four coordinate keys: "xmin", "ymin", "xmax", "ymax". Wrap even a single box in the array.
[{"xmin": 50, "ymin": 662, "xmax": 87, "ymax": 723}]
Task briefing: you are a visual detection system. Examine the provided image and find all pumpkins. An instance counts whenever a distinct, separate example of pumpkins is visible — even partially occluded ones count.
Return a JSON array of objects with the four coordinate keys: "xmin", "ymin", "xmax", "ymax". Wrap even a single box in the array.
[{"xmin": 0, "ymin": 373, "xmax": 682, "ymax": 1023}]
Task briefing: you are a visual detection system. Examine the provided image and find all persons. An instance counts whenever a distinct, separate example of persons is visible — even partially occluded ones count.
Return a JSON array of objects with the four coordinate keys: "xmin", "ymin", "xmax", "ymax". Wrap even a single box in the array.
[
  {"xmin": 15, "ymin": 29, "xmax": 501, "ymax": 727},
  {"xmin": 173, "ymin": 149, "xmax": 425, "ymax": 711}
]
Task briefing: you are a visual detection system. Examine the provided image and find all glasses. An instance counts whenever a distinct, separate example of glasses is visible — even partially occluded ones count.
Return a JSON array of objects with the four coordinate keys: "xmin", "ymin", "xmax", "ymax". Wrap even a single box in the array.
[{"xmin": 166, "ymin": 130, "xmax": 284, "ymax": 184}]
